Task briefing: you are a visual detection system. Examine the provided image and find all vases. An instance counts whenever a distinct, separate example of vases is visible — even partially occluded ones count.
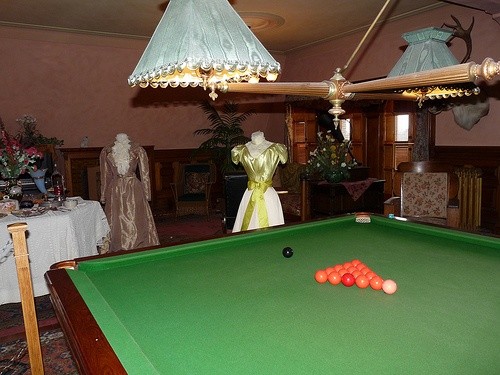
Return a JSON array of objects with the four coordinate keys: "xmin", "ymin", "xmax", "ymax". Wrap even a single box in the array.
[
  {"xmin": 325, "ymin": 171, "xmax": 344, "ymax": 183},
  {"xmin": 6, "ymin": 176, "xmax": 23, "ymax": 200}
]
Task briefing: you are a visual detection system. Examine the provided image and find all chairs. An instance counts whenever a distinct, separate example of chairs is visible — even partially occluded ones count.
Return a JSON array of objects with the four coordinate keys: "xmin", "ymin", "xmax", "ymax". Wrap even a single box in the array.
[
  {"xmin": 384, "ymin": 161, "xmax": 461, "ymax": 229},
  {"xmin": 170, "ymin": 161, "xmax": 216, "ymax": 222}
]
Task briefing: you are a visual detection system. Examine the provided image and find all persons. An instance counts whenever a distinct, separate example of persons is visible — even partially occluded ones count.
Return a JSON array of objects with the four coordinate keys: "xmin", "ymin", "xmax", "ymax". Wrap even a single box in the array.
[
  {"xmin": 99, "ymin": 133, "xmax": 160, "ymax": 254},
  {"xmin": 231, "ymin": 132, "xmax": 288, "ymax": 233}
]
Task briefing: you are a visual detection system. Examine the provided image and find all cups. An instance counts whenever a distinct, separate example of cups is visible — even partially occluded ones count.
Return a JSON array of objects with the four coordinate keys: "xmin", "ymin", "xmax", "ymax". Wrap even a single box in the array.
[{"xmin": 62, "ymin": 200, "xmax": 78, "ymax": 208}]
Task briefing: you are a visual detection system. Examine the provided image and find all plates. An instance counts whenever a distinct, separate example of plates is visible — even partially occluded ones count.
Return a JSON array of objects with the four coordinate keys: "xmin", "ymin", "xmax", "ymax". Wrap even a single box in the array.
[
  {"xmin": 0, "ymin": 213, "xmax": 8, "ymax": 220},
  {"xmin": 12, "ymin": 210, "xmax": 48, "ymax": 218},
  {"xmin": 62, "ymin": 207, "xmax": 78, "ymax": 210}
]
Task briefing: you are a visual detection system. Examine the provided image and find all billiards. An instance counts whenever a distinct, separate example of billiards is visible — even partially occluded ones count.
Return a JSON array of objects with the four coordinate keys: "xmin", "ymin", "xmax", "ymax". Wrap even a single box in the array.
[
  {"xmin": 355, "ymin": 275, "xmax": 370, "ymax": 289},
  {"xmin": 370, "ymin": 276, "xmax": 383, "ymax": 291},
  {"xmin": 328, "ymin": 272, "xmax": 342, "ymax": 285},
  {"xmin": 325, "ymin": 259, "xmax": 377, "ymax": 279},
  {"xmin": 315, "ymin": 270, "xmax": 328, "ymax": 283},
  {"xmin": 383, "ymin": 280, "xmax": 398, "ymax": 295},
  {"xmin": 282, "ymin": 247, "xmax": 294, "ymax": 258},
  {"xmin": 342, "ymin": 273, "xmax": 355, "ymax": 287}
]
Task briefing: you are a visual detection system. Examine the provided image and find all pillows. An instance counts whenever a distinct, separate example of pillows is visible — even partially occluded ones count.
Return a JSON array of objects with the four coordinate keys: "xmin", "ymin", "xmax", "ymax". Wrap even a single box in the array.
[{"xmin": 184, "ymin": 173, "xmax": 210, "ymax": 194}]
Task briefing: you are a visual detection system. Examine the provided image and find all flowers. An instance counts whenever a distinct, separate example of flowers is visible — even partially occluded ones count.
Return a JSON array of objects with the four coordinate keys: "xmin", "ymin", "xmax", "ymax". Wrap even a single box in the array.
[
  {"xmin": 308, "ymin": 130, "xmax": 357, "ymax": 174},
  {"xmin": 0, "ymin": 121, "xmax": 43, "ymax": 179}
]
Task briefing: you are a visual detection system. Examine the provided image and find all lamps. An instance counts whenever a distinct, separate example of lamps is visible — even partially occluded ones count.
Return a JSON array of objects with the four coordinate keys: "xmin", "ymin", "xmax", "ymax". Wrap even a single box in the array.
[
  {"xmin": 386, "ymin": 27, "xmax": 479, "ymax": 108},
  {"xmin": 128, "ymin": 1, "xmax": 280, "ymax": 101}
]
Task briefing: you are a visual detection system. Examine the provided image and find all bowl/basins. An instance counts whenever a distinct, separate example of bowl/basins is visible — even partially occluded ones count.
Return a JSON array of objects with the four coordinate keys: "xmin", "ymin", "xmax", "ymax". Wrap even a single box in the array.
[{"xmin": 0, "ymin": 195, "xmax": 19, "ymax": 213}]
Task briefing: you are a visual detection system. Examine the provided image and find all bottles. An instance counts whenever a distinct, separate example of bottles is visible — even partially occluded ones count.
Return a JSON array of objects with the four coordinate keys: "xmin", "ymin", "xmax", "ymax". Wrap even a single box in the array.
[{"xmin": 52, "ymin": 175, "xmax": 63, "ymax": 195}]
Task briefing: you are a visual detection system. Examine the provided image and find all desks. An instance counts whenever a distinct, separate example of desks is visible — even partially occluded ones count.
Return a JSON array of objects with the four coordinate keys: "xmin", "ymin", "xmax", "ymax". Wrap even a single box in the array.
[
  {"xmin": 42, "ymin": 212, "xmax": 500, "ymax": 374},
  {"xmin": 0, "ymin": 201, "xmax": 111, "ymax": 344},
  {"xmin": 307, "ymin": 179, "xmax": 387, "ymax": 217}
]
